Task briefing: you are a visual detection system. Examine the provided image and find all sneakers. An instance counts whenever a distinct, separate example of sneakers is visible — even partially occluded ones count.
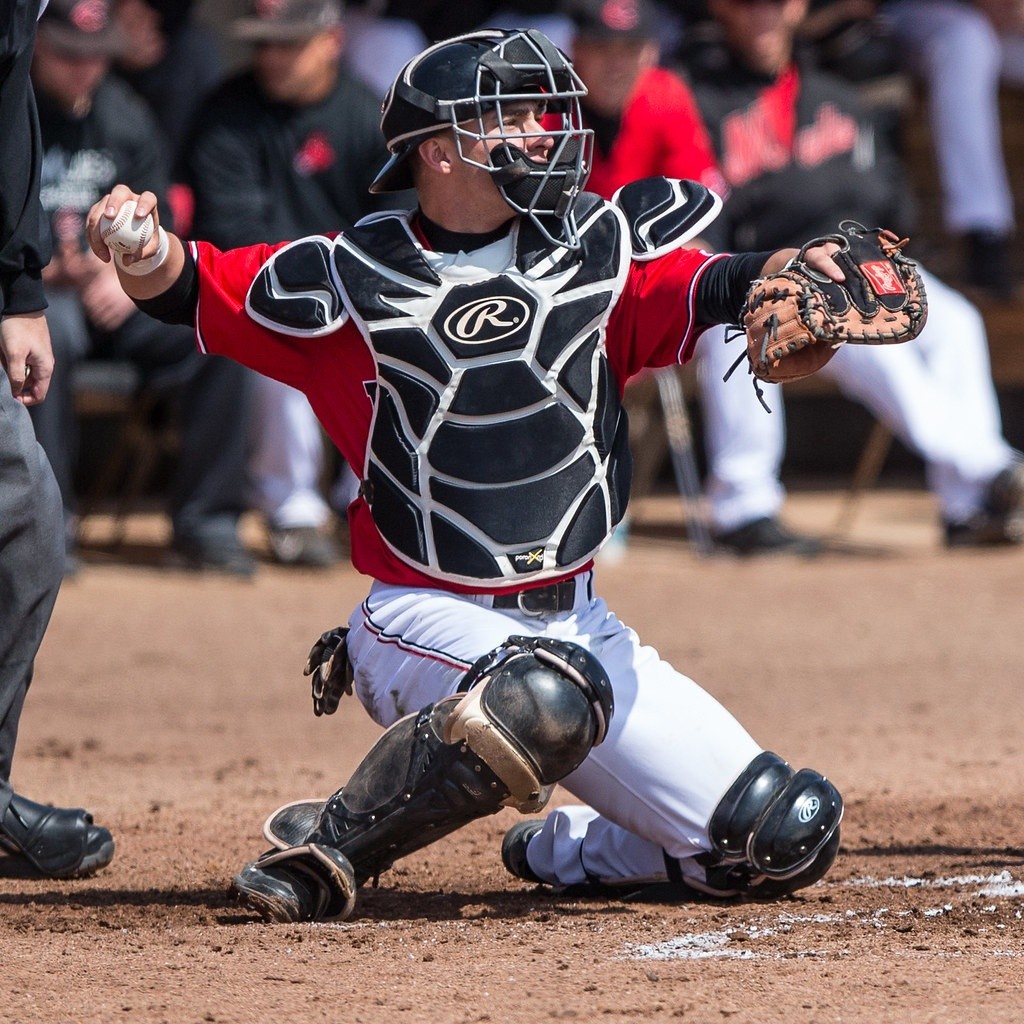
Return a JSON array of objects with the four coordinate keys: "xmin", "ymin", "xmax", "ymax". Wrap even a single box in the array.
[
  {"xmin": 501, "ymin": 820, "xmax": 552, "ymax": 884},
  {"xmin": 232, "ymin": 860, "xmax": 328, "ymax": 925}
]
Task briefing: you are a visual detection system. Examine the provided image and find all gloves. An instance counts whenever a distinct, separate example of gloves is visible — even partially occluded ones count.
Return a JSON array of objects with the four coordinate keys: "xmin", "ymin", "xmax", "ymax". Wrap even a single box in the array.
[{"xmin": 303, "ymin": 627, "xmax": 354, "ymax": 717}]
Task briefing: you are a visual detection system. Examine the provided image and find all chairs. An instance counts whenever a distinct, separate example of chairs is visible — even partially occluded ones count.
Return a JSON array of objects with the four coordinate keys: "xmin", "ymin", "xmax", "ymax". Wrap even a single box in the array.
[{"xmin": 68, "ymin": 362, "xmax": 891, "ymax": 562}]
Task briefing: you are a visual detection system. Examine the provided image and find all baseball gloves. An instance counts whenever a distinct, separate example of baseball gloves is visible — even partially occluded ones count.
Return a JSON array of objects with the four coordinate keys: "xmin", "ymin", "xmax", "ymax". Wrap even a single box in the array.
[{"xmin": 743, "ymin": 226, "xmax": 926, "ymax": 385}]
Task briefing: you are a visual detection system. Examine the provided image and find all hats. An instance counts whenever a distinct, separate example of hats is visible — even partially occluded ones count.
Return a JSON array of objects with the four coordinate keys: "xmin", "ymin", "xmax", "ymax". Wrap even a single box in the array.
[
  {"xmin": 568, "ymin": 0, "xmax": 684, "ymax": 43},
  {"xmin": 31, "ymin": 0, "xmax": 126, "ymax": 59},
  {"xmin": 220, "ymin": 0, "xmax": 340, "ymax": 40}
]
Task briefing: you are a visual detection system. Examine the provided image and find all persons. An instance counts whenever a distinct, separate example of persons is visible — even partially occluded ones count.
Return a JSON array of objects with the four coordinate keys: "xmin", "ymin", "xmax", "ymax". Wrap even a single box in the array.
[
  {"xmin": 86, "ymin": 28, "xmax": 926, "ymax": 938},
  {"xmin": 0, "ymin": 0, "xmax": 113, "ymax": 880},
  {"xmin": 28, "ymin": 0, "xmax": 1024, "ymax": 573}
]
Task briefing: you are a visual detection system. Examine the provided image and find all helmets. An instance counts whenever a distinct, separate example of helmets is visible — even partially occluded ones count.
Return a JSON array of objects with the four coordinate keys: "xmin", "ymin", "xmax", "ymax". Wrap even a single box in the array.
[{"xmin": 370, "ymin": 27, "xmax": 571, "ymax": 194}]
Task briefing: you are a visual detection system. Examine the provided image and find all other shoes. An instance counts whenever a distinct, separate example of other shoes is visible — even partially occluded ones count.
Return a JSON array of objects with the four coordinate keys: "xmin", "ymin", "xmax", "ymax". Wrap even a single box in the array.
[
  {"xmin": 943, "ymin": 459, "xmax": 1024, "ymax": 547},
  {"xmin": 959, "ymin": 225, "xmax": 1010, "ymax": 296},
  {"xmin": 710, "ymin": 517, "xmax": 807, "ymax": 559},
  {"xmin": 0, "ymin": 822, "xmax": 113, "ymax": 878},
  {"xmin": 265, "ymin": 525, "xmax": 331, "ymax": 567},
  {"xmin": 171, "ymin": 531, "xmax": 255, "ymax": 579}
]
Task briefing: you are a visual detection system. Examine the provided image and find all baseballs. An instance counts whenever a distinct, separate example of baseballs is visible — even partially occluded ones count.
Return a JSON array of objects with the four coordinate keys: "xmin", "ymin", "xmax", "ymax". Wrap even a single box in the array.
[{"xmin": 100, "ymin": 199, "xmax": 155, "ymax": 255}]
